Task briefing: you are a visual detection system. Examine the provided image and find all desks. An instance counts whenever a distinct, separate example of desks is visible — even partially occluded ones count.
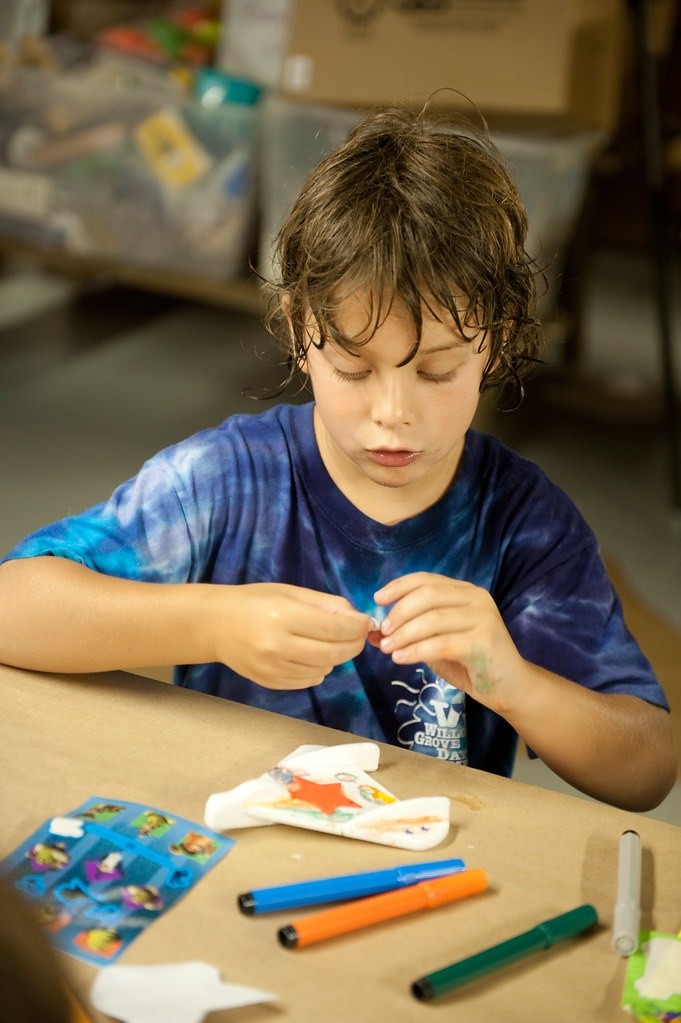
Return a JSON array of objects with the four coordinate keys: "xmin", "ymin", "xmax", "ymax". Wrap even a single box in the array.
[{"xmin": 2, "ymin": 668, "xmax": 679, "ymax": 1023}]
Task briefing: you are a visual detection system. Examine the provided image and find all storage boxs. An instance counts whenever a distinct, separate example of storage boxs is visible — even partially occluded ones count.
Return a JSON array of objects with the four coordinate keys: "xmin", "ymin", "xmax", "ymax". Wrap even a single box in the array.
[
  {"xmin": 284, "ymin": 1, "xmax": 620, "ymax": 130},
  {"xmin": 4, "ymin": 52, "xmax": 256, "ymax": 282},
  {"xmin": 256, "ymin": 96, "xmax": 578, "ymax": 325}
]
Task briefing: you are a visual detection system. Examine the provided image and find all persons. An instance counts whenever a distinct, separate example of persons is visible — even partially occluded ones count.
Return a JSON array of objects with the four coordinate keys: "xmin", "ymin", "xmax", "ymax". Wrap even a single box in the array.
[
  {"xmin": 0, "ymin": 865, "xmax": 89, "ymax": 1022},
  {"xmin": 0, "ymin": 79, "xmax": 681, "ymax": 813}
]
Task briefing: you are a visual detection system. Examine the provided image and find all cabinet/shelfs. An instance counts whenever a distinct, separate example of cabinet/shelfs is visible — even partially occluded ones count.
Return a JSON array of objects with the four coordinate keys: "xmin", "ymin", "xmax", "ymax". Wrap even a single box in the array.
[{"xmin": 3, "ymin": 8, "xmax": 679, "ymax": 507}]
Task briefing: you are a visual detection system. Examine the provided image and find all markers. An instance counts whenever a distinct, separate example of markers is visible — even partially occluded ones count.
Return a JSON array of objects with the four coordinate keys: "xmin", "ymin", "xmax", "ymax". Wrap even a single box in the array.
[
  {"xmin": 610, "ymin": 829, "xmax": 643, "ymax": 957},
  {"xmin": 237, "ymin": 858, "xmax": 466, "ymax": 917},
  {"xmin": 411, "ymin": 903, "xmax": 599, "ymax": 1003},
  {"xmin": 277, "ymin": 869, "xmax": 489, "ymax": 951}
]
